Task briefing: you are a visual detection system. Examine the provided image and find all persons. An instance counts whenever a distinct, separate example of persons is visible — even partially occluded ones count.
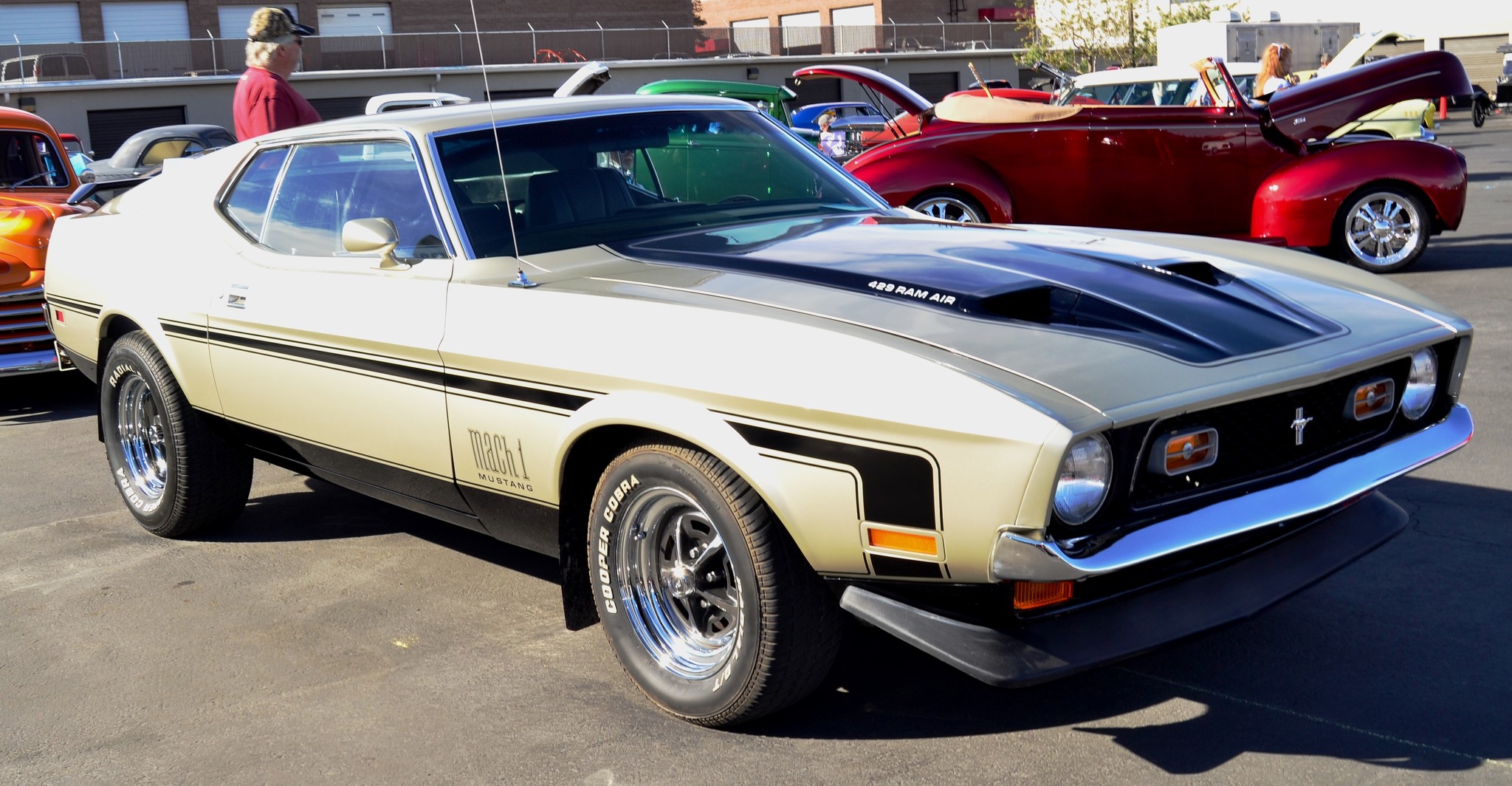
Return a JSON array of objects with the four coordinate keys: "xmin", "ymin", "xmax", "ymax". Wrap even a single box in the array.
[
  {"xmin": 231, "ymin": 5, "xmax": 323, "ymax": 144},
  {"xmin": 818, "ymin": 113, "xmax": 837, "ymax": 134},
  {"xmin": 598, "ymin": 149, "xmax": 639, "ymax": 186},
  {"xmin": 1236, "ymin": 57, "xmax": 1264, "ymax": 100},
  {"xmin": 1249, "ymin": 42, "xmax": 1300, "ymax": 100},
  {"xmin": 1315, "ymin": 52, "xmax": 1333, "ymax": 76},
  {"xmin": 1151, "ymin": 81, "xmax": 1170, "ymax": 106}
]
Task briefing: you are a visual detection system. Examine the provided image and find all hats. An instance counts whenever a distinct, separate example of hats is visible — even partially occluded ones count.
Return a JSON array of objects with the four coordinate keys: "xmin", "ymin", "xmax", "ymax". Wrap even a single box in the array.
[
  {"xmin": 817, "ymin": 114, "xmax": 837, "ymax": 125},
  {"xmin": 243, "ymin": 7, "xmax": 315, "ymax": 37}
]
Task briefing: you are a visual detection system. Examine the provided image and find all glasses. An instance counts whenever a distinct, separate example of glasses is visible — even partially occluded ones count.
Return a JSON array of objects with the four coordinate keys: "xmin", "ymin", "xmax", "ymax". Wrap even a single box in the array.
[{"xmin": 291, "ymin": 37, "xmax": 305, "ymax": 47}]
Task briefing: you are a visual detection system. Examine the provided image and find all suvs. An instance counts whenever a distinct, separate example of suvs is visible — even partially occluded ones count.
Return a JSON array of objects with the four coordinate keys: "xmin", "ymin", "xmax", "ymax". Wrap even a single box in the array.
[
  {"xmin": 1030, "ymin": 27, "xmax": 1444, "ymax": 141},
  {"xmin": 2, "ymin": 53, "xmax": 93, "ymax": 108},
  {"xmin": 628, "ymin": 77, "xmax": 817, "ymax": 204}
]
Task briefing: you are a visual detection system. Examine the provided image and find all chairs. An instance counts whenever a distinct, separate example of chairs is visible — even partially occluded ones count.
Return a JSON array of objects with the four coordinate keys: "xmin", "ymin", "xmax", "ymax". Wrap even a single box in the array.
[{"xmin": 522, "ymin": 166, "xmax": 638, "ymax": 247}]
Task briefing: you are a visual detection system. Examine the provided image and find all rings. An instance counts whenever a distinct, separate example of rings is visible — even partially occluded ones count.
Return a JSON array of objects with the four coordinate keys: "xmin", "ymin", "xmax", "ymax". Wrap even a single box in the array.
[{"xmin": 1295, "ymin": 76, "xmax": 1298, "ymax": 77}]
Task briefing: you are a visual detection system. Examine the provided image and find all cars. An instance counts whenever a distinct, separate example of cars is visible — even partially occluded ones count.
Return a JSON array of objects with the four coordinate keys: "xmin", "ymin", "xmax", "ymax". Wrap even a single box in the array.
[
  {"xmin": 796, "ymin": 50, "xmax": 1480, "ymax": 271},
  {"xmin": 83, "ymin": 123, "xmax": 236, "ymax": 204},
  {"xmin": 33, "ymin": 108, "xmax": 1471, "ymax": 726},
  {"xmin": 789, "ymin": 94, "xmax": 888, "ymax": 146},
  {"xmin": 1, "ymin": 105, "xmax": 93, "ymax": 387}
]
